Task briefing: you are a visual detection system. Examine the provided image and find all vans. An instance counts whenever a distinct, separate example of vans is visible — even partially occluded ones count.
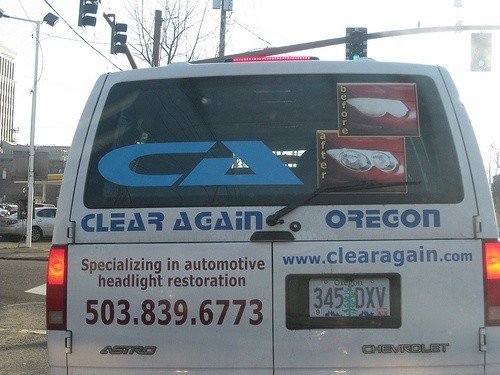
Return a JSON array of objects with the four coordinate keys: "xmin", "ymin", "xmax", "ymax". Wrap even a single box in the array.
[{"xmin": 48, "ymin": 61, "xmax": 499, "ymax": 374}]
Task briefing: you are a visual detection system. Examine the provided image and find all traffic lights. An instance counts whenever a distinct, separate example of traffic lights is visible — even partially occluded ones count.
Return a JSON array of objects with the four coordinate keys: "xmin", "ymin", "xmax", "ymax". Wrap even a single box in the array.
[
  {"xmin": 469, "ymin": 31, "xmax": 492, "ymax": 70},
  {"xmin": 109, "ymin": 22, "xmax": 128, "ymax": 53},
  {"xmin": 78, "ymin": 0, "xmax": 99, "ymax": 26},
  {"xmin": 343, "ymin": 28, "xmax": 367, "ymax": 60}
]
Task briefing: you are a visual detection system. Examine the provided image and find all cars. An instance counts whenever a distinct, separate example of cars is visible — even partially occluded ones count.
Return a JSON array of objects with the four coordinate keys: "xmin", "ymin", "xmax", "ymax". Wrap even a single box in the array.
[
  {"xmin": 1, "ymin": 207, "xmax": 59, "ymax": 242},
  {"xmin": 1, "ymin": 199, "xmax": 55, "ymax": 216}
]
